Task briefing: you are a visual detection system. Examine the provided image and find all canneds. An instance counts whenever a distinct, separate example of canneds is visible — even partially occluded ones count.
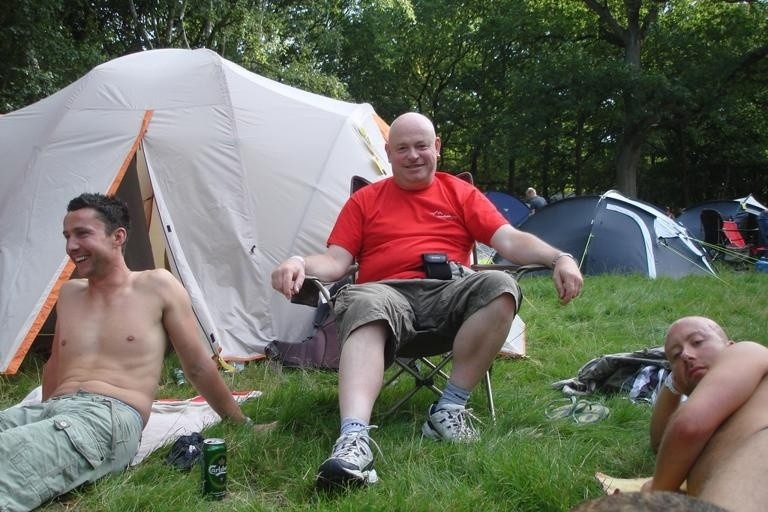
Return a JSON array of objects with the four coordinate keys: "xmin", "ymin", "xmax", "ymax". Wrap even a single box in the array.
[{"xmin": 201, "ymin": 437, "xmax": 226, "ymax": 501}]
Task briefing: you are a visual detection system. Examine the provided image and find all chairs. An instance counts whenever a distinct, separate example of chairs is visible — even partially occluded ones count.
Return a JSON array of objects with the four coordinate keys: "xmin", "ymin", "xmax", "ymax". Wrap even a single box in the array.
[
  {"xmin": 290, "ymin": 172, "xmax": 549, "ymax": 433},
  {"xmin": 721, "ymin": 220, "xmax": 768, "ymax": 272}
]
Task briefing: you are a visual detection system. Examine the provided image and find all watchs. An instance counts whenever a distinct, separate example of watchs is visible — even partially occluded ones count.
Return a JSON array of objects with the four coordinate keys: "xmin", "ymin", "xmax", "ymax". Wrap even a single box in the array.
[{"xmin": 662, "ymin": 370, "xmax": 684, "ymax": 399}]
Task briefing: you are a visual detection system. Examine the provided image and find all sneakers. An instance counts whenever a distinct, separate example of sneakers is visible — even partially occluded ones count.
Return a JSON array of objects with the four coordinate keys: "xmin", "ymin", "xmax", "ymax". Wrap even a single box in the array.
[
  {"xmin": 318, "ymin": 425, "xmax": 383, "ymax": 484},
  {"xmin": 422, "ymin": 402, "xmax": 485, "ymax": 444}
]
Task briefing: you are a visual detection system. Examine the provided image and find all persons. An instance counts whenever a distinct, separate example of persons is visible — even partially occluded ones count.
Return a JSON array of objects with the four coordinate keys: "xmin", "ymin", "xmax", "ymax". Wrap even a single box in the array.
[
  {"xmin": 0, "ymin": 188, "xmax": 279, "ymax": 512},
  {"xmin": 524, "ymin": 186, "xmax": 548, "ymax": 217},
  {"xmin": 664, "ymin": 206, "xmax": 674, "ymax": 219},
  {"xmin": 265, "ymin": 111, "xmax": 586, "ymax": 486},
  {"xmin": 636, "ymin": 311, "xmax": 766, "ymax": 512}
]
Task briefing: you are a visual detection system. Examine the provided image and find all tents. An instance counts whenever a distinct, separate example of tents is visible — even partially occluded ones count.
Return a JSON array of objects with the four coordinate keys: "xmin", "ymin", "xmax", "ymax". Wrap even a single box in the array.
[
  {"xmin": 481, "ymin": 189, "xmax": 529, "ymax": 230},
  {"xmin": 0, "ymin": 42, "xmax": 527, "ymax": 379},
  {"xmin": 672, "ymin": 194, "xmax": 767, "ymax": 261},
  {"xmin": 484, "ymin": 188, "xmax": 718, "ymax": 279}
]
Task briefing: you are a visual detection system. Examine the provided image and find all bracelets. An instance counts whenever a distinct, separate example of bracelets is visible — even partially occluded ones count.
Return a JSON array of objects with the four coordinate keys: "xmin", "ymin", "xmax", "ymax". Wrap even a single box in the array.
[
  {"xmin": 290, "ymin": 255, "xmax": 307, "ymax": 271},
  {"xmin": 548, "ymin": 252, "xmax": 575, "ymax": 271}
]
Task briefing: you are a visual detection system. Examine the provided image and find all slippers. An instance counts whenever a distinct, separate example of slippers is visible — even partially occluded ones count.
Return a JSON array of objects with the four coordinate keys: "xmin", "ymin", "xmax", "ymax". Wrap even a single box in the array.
[
  {"xmin": 573, "ymin": 399, "xmax": 609, "ymax": 425},
  {"xmin": 545, "ymin": 396, "xmax": 585, "ymax": 424}
]
